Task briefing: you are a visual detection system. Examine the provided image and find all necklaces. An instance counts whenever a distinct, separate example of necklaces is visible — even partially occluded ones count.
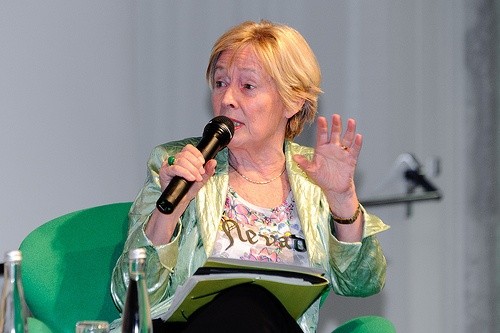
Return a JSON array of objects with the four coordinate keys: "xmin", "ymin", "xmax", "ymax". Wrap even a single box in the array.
[{"xmin": 228, "ymin": 161, "xmax": 286, "ymax": 184}]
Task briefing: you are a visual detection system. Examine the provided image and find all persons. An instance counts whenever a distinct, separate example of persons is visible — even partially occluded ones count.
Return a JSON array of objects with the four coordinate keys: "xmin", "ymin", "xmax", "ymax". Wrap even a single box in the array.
[{"xmin": 111, "ymin": 18, "xmax": 397, "ymax": 333}]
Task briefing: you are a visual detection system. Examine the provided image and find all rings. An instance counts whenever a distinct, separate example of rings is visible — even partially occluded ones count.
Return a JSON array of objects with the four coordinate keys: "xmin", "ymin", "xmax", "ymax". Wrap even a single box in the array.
[
  {"xmin": 167, "ymin": 156, "xmax": 178, "ymax": 165},
  {"xmin": 341, "ymin": 144, "xmax": 350, "ymax": 152}
]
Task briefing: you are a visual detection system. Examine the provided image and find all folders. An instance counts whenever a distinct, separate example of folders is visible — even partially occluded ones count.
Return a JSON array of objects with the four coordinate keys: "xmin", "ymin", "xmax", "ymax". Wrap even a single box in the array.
[{"xmin": 161, "ymin": 261, "xmax": 330, "ymax": 321}]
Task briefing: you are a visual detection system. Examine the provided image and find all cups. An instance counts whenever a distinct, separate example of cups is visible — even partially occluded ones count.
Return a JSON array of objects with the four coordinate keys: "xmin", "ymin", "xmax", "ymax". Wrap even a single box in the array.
[{"xmin": 75, "ymin": 320, "xmax": 110, "ymax": 333}]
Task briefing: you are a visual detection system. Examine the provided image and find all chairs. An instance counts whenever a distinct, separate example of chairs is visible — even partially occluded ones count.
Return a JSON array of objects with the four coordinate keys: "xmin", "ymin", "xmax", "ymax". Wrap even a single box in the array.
[{"xmin": 0, "ymin": 201, "xmax": 395, "ymax": 333}]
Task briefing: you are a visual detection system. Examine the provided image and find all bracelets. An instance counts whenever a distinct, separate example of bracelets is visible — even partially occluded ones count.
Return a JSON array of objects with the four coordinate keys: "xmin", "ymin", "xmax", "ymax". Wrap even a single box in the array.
[{"xmin": 329, "ymin": 203, "xmax": 361, "ymax": 224}]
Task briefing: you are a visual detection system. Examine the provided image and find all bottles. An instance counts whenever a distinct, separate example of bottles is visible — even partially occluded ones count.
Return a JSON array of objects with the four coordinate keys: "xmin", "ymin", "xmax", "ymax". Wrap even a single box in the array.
[
  {"xmin": 0, "ymin": 249, "xmax": 35, "ymax": 333},
  {"xmin": 120, "ymin": 248, "xmax": 153, "ymax": 333}
]
